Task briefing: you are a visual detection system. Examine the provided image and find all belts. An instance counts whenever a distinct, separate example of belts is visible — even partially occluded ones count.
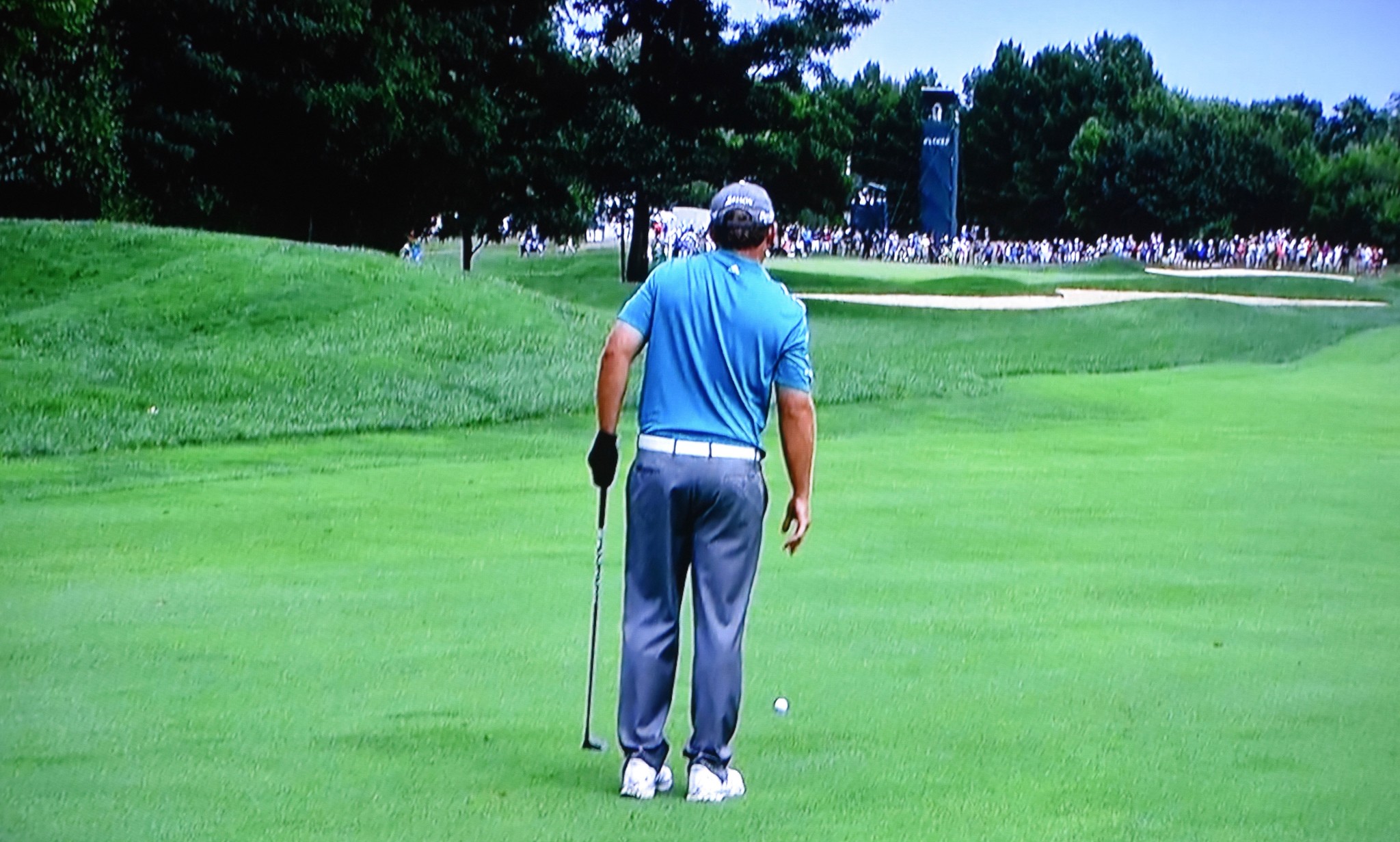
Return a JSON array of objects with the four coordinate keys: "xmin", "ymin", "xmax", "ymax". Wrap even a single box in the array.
[{"xmin": 639, "ymin": 433, "xmax": 761, "ymax": 461}]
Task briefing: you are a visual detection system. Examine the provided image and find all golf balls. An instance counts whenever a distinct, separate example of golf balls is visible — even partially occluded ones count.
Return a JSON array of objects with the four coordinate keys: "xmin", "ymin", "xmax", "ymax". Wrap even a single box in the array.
[{"xmin": 774, "ymin": 697, "xmax": 788, "ymax": 713}]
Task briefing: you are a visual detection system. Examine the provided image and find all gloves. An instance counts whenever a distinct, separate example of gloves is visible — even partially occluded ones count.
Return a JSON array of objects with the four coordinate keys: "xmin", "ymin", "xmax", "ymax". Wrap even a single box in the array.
[{"xmin": 588, "ymin": 427, "xmax": 620, "ymax": 487}]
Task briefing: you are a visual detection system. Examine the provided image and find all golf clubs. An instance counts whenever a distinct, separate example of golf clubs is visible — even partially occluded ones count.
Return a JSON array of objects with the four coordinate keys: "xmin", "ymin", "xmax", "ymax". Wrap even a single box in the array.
[{"xmin": 580, "ymin": 487, "xmax": 607, "ymax": 752}]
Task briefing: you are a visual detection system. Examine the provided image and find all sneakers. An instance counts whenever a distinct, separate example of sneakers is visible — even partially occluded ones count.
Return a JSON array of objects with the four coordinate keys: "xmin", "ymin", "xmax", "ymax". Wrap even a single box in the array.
[
  {"xmin": 685, "ymin": 764, "xmax": 745, "ymax": 804},
  {"xmin": 620, "ymin": 756, "xmax": 673, "ymax": 799}
]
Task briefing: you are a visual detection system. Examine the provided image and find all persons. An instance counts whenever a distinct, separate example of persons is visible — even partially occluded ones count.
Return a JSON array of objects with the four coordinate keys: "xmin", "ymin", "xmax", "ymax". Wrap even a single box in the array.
[
  {"xmin": 587, "ymin": 179, "xmax": 818, "ymax": 804},
  {"xmin": 765, "ymin": 219, "xmax": 1386, "ymax": 277},
  {"xmin": 500, "ymin": 218, "xmax": 576, "ymax": 257},
  {"xmin": 646, "ymin": 214, "xmax": 716, "ymax": 265},
  {"xmin": 402, "ymin": 235, "xmax": 423, "ymax": 262}
]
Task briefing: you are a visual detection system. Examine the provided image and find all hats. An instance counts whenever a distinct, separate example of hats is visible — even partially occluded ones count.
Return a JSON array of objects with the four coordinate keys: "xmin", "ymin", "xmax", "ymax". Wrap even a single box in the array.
[{"xmin": 710, "ymin": 180, "xmax": 775, "ymax": 229}]
